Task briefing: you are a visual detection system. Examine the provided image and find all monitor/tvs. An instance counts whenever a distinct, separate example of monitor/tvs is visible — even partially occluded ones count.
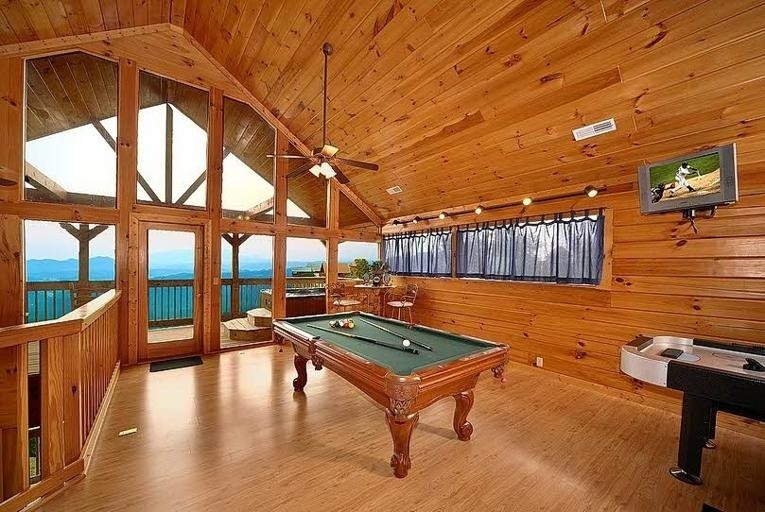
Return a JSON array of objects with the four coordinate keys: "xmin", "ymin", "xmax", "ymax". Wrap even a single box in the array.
[{"xmin": 638, "ymin": 142, "xmax": 739, "ymax": 214}]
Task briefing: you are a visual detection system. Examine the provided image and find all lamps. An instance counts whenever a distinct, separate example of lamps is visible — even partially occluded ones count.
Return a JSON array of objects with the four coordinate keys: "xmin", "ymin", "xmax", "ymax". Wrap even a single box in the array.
[
  {"xmin": 306, "ymin": 154, "xmax": 339, "ymax": 180},
  {"xmin": 392, "ymin": 182, "xmax": 601, "ymax": 233}
]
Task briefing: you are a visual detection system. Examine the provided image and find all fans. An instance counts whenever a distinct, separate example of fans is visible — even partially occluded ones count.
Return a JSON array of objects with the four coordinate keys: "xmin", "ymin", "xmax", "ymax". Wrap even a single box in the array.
[{"xmin": 260, "ymin": 41, "xmax": 382, "ymax": 186}]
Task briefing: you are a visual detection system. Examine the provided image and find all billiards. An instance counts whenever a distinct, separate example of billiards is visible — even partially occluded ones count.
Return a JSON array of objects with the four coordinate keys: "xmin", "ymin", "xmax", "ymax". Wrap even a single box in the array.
[
  {"xmin": 403, "ymin": 339, "xmax": 410, "ymax": 347},
  {"xmin": 329, "ymin": 318, "xmax": 355, "ymax": 329},
  {"xmin": 502, "ymin": 377, "xmax": 506, "ymax": 383}
]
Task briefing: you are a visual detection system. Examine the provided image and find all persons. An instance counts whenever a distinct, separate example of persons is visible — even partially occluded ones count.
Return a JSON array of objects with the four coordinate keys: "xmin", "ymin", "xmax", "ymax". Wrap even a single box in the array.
[
  {"xmin": 650, "ymin": 180, "xmax": 678, "ymax": 205},
  {"xmin": 667, "ymin": 160, "xmax": 700, "ymax": 198}
]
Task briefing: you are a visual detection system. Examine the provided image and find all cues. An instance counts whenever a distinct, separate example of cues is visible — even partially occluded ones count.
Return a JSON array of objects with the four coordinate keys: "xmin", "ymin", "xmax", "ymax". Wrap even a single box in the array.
[
  {"xmin": 306, "ymin": 323, "xmax": 419, "ymax": 355},
  {"xmin": 360, "ymin": 318, "xmax": 432, "ymax": 352}
]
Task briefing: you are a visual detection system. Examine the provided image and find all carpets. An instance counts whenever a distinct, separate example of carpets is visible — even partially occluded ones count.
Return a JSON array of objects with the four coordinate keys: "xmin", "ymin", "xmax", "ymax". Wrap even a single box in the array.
[{"xmin": 148, "ymin": 354, "xmax": 202, "ymax": 373}]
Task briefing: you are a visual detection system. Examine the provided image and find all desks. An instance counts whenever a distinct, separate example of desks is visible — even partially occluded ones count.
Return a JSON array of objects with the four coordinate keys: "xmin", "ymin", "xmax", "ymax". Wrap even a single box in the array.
[
  {"xmin": 268, "ymin": 309, "xmax": 511, "ymax": 480},
  {"xmin": 353, "ymin": 282, "xmax": 397, "ymax": 319},
  {"xmin": 620, "ymin": 331, "xmax": 764, "ymax": 482}
]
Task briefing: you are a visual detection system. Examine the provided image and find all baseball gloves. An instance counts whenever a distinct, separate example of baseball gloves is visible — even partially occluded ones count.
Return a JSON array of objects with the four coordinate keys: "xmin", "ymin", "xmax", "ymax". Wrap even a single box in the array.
[{"xmin": 668, "ymin": 183, "xmax": 675, "ymax": 188}]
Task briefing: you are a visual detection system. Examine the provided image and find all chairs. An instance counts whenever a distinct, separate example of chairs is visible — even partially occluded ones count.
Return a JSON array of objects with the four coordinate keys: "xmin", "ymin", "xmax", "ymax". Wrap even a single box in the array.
[
  {"xmin": 386, "ymin": 281, "xmax": 421, "ymax": 320},
  {"xmin": 326, "ymin": 281, "xmax": 363, "ymax": 312}
]
